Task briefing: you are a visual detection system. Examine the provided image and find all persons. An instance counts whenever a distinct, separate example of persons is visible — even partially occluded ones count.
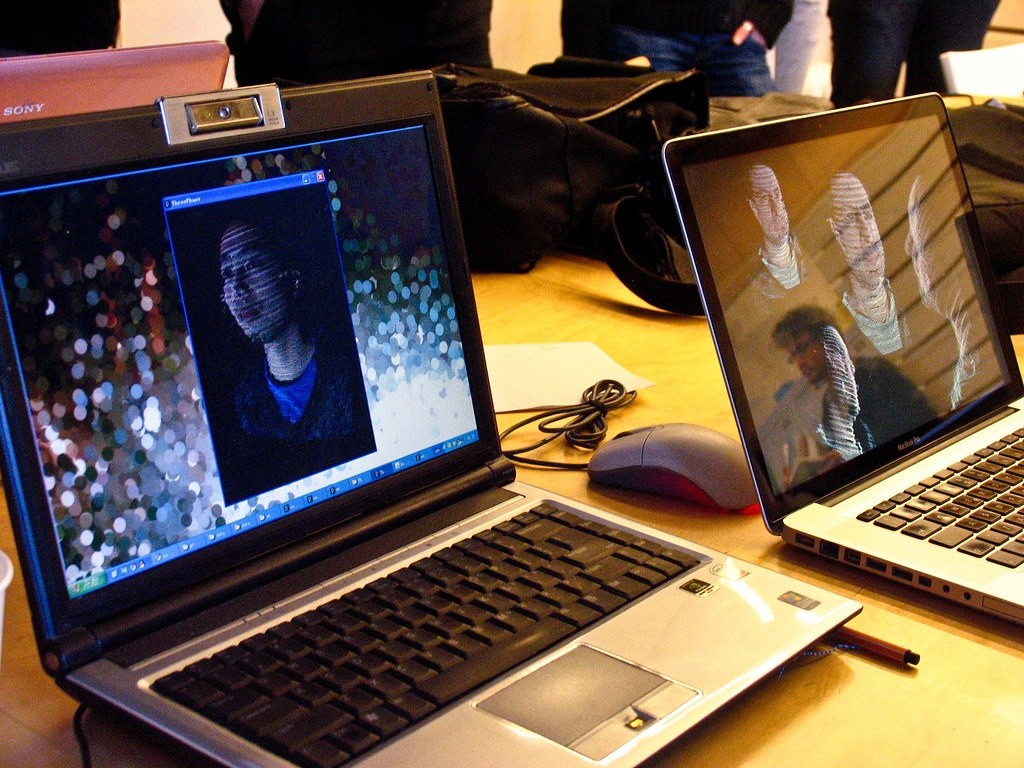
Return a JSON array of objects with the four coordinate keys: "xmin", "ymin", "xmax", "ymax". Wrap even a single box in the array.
[
  {"xmin": 219, "ymin": 0, "xmax": 494, "ymax": 91},
  {"xmin": 559, "ymin": 1, "xmax": 793, "ymax": 100},
  {"xmin": 829, "ymin": 0, "xmax": 999, "ymax": 107},
  {"xmin": 730, "ymin": 160, "xmax": 1001, "ymax": 473},
  {"xmin": 207, "ymin": 212, "xmax": 376, "ymax": 506}
]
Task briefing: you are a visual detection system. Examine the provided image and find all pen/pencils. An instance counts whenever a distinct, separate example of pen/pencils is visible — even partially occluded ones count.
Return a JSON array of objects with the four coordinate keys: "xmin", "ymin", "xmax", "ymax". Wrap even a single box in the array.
[{"xmin": 820, "ymin": 625, "xmax": 919, "ymax": 667}]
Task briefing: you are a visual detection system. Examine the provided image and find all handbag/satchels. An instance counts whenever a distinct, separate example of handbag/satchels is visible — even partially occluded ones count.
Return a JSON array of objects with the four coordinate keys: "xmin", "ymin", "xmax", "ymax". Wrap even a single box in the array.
[{"xmin": 431, "ymin": 60, "xmax": 710, "ymax": 316}]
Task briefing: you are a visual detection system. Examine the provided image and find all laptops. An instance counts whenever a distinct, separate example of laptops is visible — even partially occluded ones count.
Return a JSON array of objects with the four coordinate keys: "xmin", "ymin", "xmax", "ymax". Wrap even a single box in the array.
[
  {"xmin": 0, "ymin": 40, "xmax": 230, "ymax": 122},
  {"xmin": 0, "ymin": 70, "xmax": 864, "ymax": 768},
  {"xmin": 659, "ymin": 93, "xmax": 1024, "ymax": 626}
]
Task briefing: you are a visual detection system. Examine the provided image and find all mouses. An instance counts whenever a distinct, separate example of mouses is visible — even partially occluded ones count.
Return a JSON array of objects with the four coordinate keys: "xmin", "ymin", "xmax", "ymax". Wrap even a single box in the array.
[{"xmin": 588, "ymin": 422, "xmax": 759, "ymax": 513}]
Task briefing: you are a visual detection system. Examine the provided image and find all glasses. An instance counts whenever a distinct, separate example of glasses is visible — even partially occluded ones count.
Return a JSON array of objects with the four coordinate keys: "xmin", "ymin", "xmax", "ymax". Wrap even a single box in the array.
[{"xmin": 786, "ymin": 344, "xmax": 812, "ymax": 363}]
